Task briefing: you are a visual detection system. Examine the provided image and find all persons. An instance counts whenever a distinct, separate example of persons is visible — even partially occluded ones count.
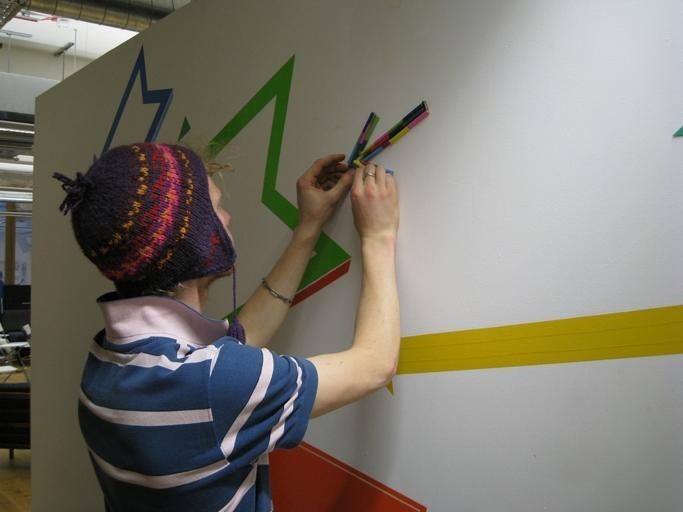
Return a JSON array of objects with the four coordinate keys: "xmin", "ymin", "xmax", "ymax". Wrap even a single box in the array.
[{"xmin": 50, "ymin": 134, "xmax": 402, "ymax": 511}]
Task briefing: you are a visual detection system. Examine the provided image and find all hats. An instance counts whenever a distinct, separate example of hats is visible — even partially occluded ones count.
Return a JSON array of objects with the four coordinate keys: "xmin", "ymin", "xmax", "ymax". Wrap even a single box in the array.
[{"xmin": 68, "ymin": 139, "xmax": 238, "ymax": 291}]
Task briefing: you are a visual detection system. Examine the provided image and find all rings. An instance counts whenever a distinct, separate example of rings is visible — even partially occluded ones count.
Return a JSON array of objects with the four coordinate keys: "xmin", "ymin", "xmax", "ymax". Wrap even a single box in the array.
[{"xmin": 364, "ymin": 171, "xmax": 375, "ymax": 178}]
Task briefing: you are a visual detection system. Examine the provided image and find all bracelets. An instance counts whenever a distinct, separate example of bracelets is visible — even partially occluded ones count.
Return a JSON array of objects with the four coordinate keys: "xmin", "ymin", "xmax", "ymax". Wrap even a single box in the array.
[{"xmin": 259, "ymin": 278, "xmax": 292, "ymax": 307}]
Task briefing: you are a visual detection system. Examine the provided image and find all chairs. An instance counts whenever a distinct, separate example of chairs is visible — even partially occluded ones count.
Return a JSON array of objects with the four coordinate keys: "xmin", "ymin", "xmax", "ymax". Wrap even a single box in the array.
[{"xmin": 0, "ymin": 310, "xmax": 31, "ymax": 382}]
